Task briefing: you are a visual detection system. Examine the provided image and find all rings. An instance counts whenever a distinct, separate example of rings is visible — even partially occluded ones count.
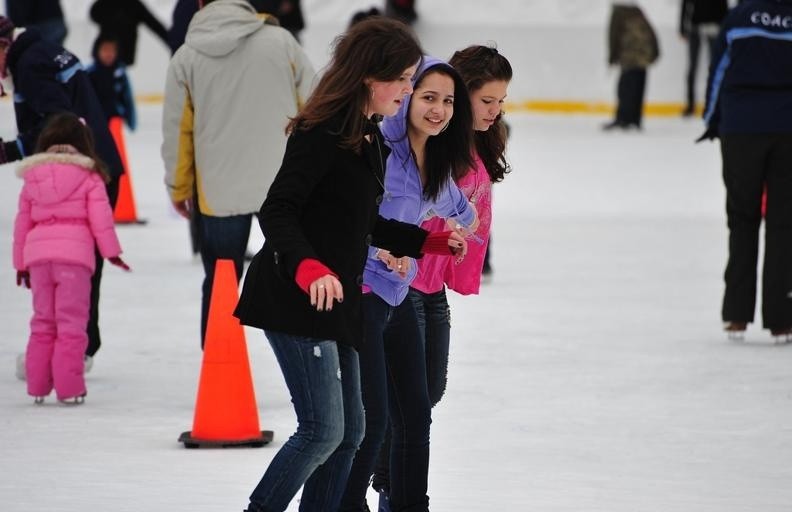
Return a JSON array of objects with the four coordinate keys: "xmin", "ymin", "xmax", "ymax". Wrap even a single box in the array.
[
  {"xmin": 317, "ymin": 285, "xmax": 325, "ymax": 290},
  {"xmin": 397, "ymin": 264, "xmax": 401, "ymax": 270}
]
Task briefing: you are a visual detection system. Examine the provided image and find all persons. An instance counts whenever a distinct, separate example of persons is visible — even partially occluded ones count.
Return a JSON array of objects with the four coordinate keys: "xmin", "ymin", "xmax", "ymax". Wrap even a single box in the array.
[
  {"xmin": 5, "ymin": 0, "xmax": 305, "ymax": 132},
  {"xmin": 677, "ymin": 0, "xmax": 730, "ymax": 121},
  {"xmin": 337, "ymin": 56, "xmax": 477, "ymax": 512},
  {"xmin": 690, "ymin": 0, "xmax": 791, "ymax": 339},
  {"xmin": 8, "ymin": 110, "xmax": 132, "ymax": 406},
  {"xmin": 0, "ymin": 14, "xmax": 127, "ymax": 382},
  {"xmin": 373, "ymin": 41, "xmax": 516, "ymax": 510},
  {"xmin": 349, "ymin": 1, "xmax": 419, "ymax": 29},
  {"xmin": 598, "ymin": 0, "xmax": 660, "ymax": 132},
  {"xmin": 160, "ymin": 0, "xmax": 322, "ymax": 357},
  {"xmin": 223, "ymin": 17, "xmax": 467, "ymax": 512}
]
